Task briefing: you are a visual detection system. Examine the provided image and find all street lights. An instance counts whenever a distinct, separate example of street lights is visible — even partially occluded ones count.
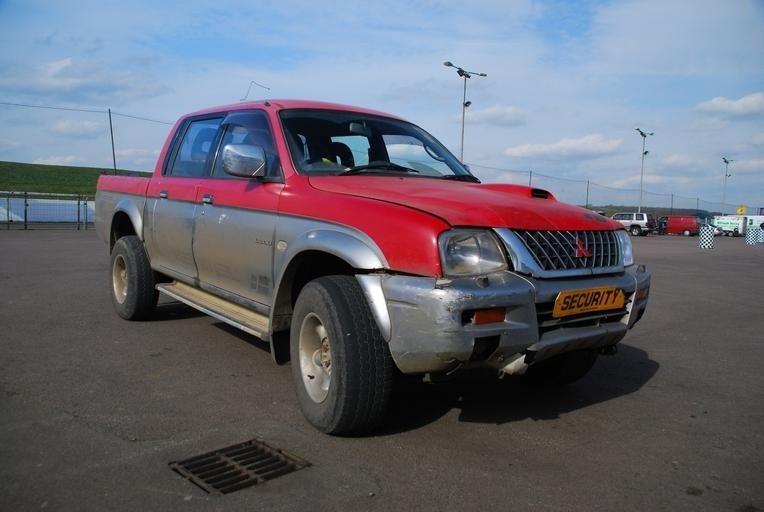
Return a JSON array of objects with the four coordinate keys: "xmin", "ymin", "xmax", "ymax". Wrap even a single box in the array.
[
  {"xmin": 632, "ymin": 123, "xmax": 654, "ymax": 213},
  {"xmin": 443, "ymin": 60, "xmax": 488, "ymax": 167},
  {"xmin": 721, "ymin": 156, "xmax": 735, "ymax": 216}
]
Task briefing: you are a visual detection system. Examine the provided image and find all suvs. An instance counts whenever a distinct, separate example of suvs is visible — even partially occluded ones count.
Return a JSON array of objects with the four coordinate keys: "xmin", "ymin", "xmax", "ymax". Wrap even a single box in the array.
[{"xmin": 608, "ymin": 212, "xmax": 656, "ymax": 237}]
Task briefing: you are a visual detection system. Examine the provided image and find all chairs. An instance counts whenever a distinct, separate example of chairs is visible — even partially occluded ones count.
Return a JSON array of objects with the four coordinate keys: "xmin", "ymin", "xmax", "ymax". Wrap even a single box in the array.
[{"xmin": 191, "ymin": 125, "xmax": 355, "ymax": 178}]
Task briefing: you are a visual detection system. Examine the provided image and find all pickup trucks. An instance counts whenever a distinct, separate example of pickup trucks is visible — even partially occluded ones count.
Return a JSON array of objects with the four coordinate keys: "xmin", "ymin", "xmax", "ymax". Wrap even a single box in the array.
[{"xmin": 92, "ymin": 98, "xmax": 653, "ymax": 439}]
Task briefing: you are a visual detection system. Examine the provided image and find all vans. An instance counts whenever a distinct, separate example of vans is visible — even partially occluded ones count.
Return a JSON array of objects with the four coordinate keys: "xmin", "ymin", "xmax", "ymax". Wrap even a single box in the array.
[{"xmin": 657, "ymin": 215, "xmax": 701, "ymax": 237}]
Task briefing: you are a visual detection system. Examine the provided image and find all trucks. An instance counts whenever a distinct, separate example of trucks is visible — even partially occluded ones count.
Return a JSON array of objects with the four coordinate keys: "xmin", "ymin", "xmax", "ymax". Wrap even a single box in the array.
[{"xmin": 705, "ymin": 215, "xmax": 764, "ymax": 237}]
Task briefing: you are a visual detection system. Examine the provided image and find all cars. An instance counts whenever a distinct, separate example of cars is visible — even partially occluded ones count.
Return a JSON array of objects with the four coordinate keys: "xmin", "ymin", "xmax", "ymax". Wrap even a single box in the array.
[{"xmin": 700, "ymin": 223, "xmax": 723, "ymax": 237}]
